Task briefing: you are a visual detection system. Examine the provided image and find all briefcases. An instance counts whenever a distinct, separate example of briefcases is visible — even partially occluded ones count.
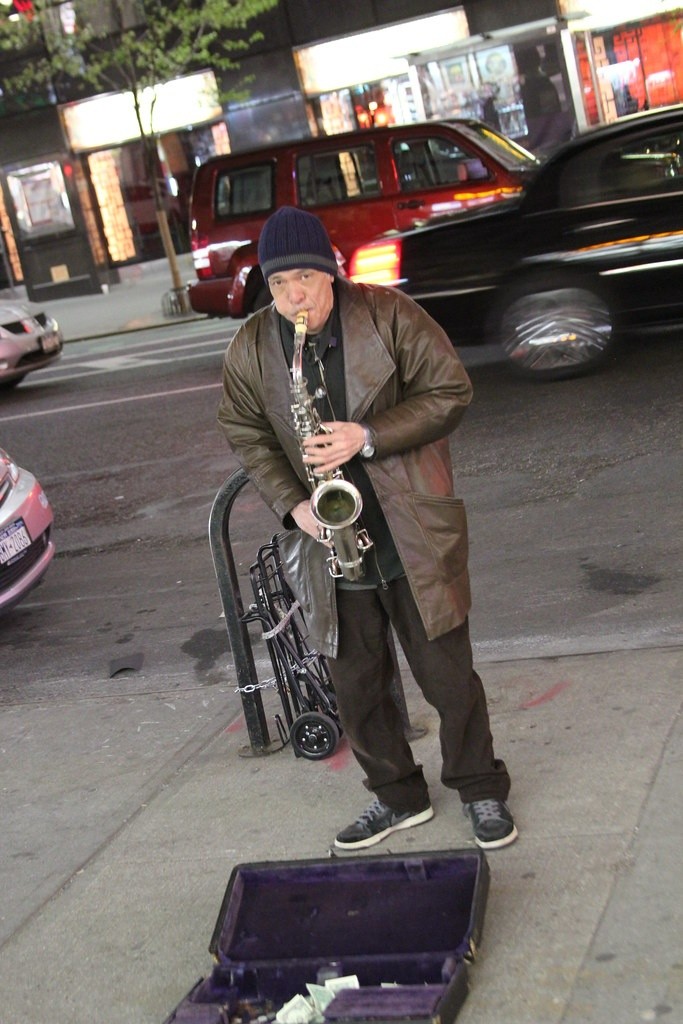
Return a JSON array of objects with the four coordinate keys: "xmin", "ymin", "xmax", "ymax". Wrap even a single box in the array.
[{"xmin": 167, "ymin": 848, "xmax": 488, "ymax": 1024}]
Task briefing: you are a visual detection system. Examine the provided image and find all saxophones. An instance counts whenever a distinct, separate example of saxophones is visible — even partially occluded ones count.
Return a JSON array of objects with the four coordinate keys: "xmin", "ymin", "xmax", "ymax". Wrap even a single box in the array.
[{"xmin": 288, "ymin": 310, "xmax": 375, "ymax": 583}]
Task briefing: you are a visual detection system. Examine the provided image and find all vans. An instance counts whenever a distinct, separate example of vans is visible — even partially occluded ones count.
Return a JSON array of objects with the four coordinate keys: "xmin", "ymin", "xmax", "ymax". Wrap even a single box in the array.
[{"xmin": 186, "ymin": 116, "xmax": 543, "ymax": 318}]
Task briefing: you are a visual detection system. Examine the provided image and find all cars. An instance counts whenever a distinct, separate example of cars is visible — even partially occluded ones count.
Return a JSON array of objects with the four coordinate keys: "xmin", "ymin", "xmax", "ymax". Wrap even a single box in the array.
[
  {"xmin": 1, "ymin": 299, "xmax": 66, "ymax": 396},
  {"xmin": 0, "ymin": 449, "xmax": 58, "ymax": 612},
  {"xmin": 461, "ymin": 103, "xmax": 683, "ymax": 380}
]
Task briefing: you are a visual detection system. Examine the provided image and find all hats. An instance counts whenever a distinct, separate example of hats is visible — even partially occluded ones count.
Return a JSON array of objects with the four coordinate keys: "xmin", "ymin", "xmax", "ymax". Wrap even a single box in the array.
[{"xmin": 257, "ymin": 207, "xmax": 338, "ymax": 282}]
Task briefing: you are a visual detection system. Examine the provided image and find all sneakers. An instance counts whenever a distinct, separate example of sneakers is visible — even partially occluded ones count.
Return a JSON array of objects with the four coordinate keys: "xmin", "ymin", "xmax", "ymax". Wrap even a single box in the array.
[
  {"xmin": 462, "ymin": 796, "xmax": 515, "ymax": 848},
  {"xmin": 332, "ymin": 799, "xmax": 435, "ymax": 848}
]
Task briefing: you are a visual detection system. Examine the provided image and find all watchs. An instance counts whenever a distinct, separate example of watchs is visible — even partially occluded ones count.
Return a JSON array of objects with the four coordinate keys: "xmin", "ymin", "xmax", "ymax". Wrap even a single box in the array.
[{"xmin": 359, "ymin": 424, "xmax": 374, "ymax": 458}]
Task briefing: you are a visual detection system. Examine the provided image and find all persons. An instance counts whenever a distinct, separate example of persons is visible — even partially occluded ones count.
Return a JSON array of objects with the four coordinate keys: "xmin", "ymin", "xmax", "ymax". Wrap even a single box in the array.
[
  {"xmin": 518, "ymin": 59, "xmax": 561, "ymax": 148},
  {"xmin": 216, "ymin": 207, "xmax": 519, "ymax": 849}
]
